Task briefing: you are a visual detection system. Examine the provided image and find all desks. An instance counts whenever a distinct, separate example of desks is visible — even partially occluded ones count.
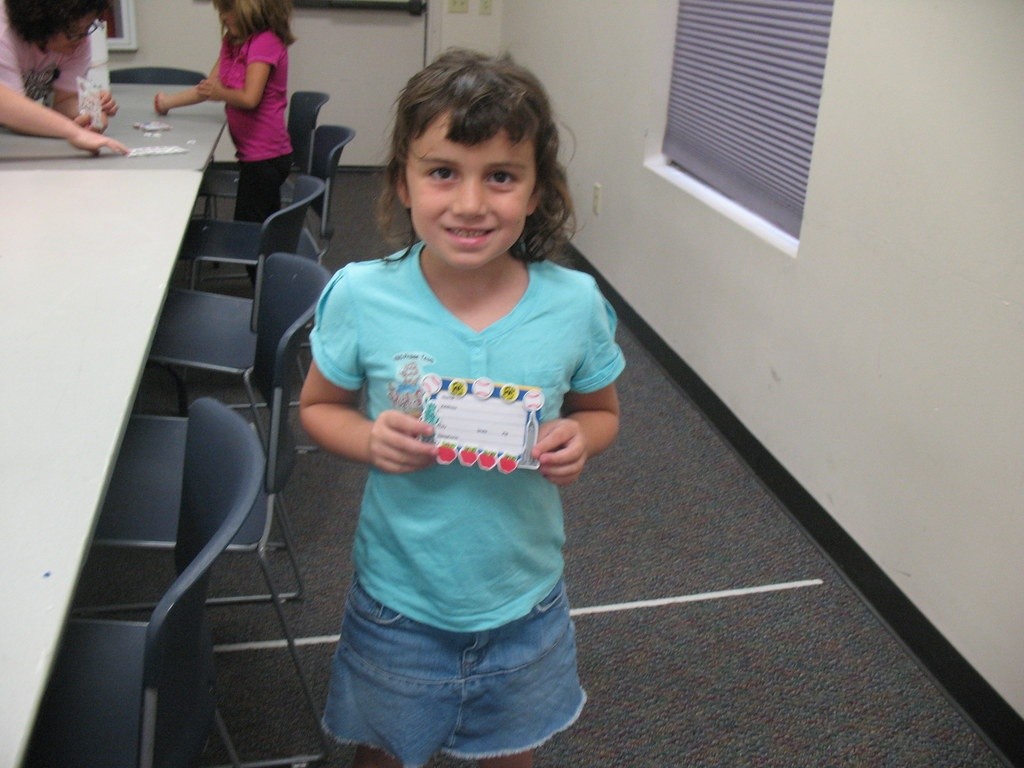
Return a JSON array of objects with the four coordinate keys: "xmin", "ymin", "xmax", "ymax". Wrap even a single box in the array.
[{"xmin": 0, "ymin": 83, "xmax": 229, "ymax": 768}]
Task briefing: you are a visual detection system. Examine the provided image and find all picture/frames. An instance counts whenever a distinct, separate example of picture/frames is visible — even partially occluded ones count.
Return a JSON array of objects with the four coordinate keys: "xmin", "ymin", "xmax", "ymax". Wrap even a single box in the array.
[{"xmin": 103, "ymin": 0, "xmax": 138, "ymax": 52}]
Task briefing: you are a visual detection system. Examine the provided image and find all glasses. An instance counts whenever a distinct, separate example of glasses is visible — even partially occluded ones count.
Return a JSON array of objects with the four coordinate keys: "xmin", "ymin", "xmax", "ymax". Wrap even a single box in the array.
[{"xmin": 62, "ymin": 22, "xmax": 98, "ymax": 43}]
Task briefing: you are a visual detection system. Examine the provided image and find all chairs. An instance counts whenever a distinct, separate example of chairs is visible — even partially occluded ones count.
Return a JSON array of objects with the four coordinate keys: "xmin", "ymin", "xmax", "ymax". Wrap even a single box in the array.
[{"xmin": 20, "ymin": 69, "xmax": 355, "ymax": 768}]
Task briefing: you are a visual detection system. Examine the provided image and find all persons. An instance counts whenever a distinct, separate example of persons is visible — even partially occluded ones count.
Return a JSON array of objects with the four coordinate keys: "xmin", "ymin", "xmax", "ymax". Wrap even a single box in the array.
[
  {"xmin": 298, "ymin": 44, "xmax": 625, "ymax": 768},
  {"xmin": 154, "ymin": 0, "xmax": 295, "ymax": 288},
  {"xmin": 0, "ymin": 0, "xmax": 132, "ymax": 157}
]
